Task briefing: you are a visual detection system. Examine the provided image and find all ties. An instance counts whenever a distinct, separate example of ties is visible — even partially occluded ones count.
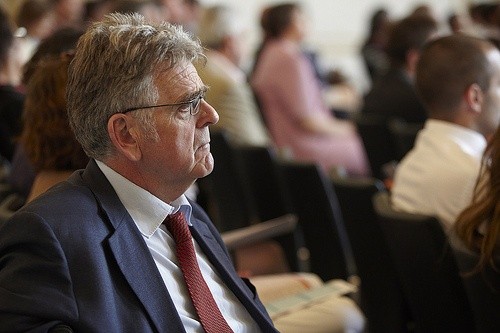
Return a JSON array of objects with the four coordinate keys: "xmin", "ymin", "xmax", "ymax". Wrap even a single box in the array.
[{"xmin": 161, "ymin": 210, "xmax": 234, "ymax": 332}]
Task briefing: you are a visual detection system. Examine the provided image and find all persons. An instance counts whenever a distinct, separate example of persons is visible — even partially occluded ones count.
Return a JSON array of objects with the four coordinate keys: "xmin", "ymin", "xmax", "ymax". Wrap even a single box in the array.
[
  {"xmin": 20, "ymin": 51, "xmax": 367, "ymax": 333},
  {"xmin": 250, "ymin": 3, "xmax": 372, "ymax": 178},
  {"xmin": 363, "ymin": 0, "xmax": 500, "ymax": 180},
  {"xmin": 389, "ymin": 32, "xmax": 500, "ymax": 277},
  {"xmin": 0, "ymin": 0, "xmax": 273, "ymax": 224},
  {"xmin": 0, "ymin": 12, "xmax": 278, "ymax": 333}
]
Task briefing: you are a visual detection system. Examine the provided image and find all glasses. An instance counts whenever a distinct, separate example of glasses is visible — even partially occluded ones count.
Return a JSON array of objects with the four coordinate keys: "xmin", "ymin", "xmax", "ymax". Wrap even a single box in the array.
[{"xmin": 122, "ymin": 92, "xmax": 205, "ymax": 115}]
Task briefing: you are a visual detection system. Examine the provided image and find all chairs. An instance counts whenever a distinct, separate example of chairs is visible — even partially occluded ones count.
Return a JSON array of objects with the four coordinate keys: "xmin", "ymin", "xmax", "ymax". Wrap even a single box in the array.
[{"xmin": 209, "ymin": 133, "xmax": 460, "ymax": 333}]
